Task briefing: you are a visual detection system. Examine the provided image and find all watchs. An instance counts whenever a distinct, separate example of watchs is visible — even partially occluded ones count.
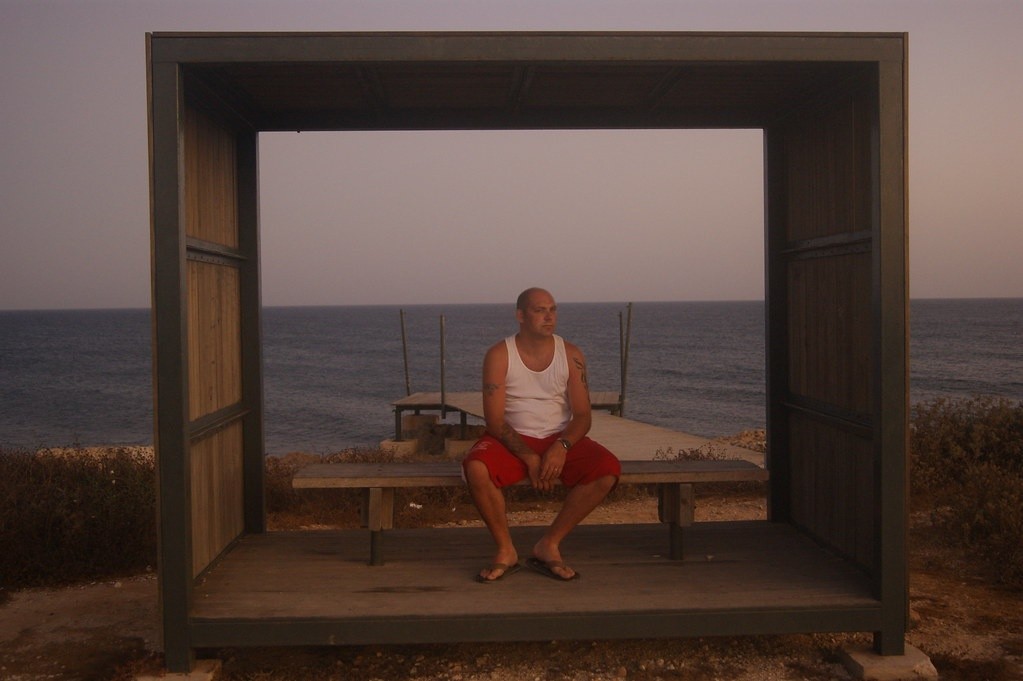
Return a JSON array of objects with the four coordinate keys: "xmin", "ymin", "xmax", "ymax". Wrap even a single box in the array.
[{"xmin": 557, "ymin": 438, "xmax": 572, "ymax": 450}]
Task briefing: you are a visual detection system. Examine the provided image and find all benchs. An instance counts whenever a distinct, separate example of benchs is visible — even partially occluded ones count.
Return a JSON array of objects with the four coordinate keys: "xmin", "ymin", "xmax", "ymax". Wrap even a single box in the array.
[{"xmin": 295, "ymin": 459, "xmax": 771, "ymax": 567}]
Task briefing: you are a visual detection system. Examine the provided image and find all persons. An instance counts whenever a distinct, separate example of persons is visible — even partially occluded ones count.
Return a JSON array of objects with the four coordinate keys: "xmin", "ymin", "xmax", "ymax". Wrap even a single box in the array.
[{"xmin": 463, "ymin": 288, "xmax": 621, "ymax": 583}]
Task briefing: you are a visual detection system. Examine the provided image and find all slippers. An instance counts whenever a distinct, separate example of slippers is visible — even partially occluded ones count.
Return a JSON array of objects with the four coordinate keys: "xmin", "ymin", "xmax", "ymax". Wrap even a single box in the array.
[
  {"xmin": 476, "ymin": 560, "xmax": 522, "ymax": 584},
  {"xmin": 526, "ymin": 557, "xmax": 579, "ymax": 582}
]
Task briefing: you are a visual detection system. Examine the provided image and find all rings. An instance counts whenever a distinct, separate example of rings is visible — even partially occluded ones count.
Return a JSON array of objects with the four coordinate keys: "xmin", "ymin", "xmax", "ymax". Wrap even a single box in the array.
[{"xmin": 554, "ymin": 468, "xmax": 558, "ymax": 472}]
